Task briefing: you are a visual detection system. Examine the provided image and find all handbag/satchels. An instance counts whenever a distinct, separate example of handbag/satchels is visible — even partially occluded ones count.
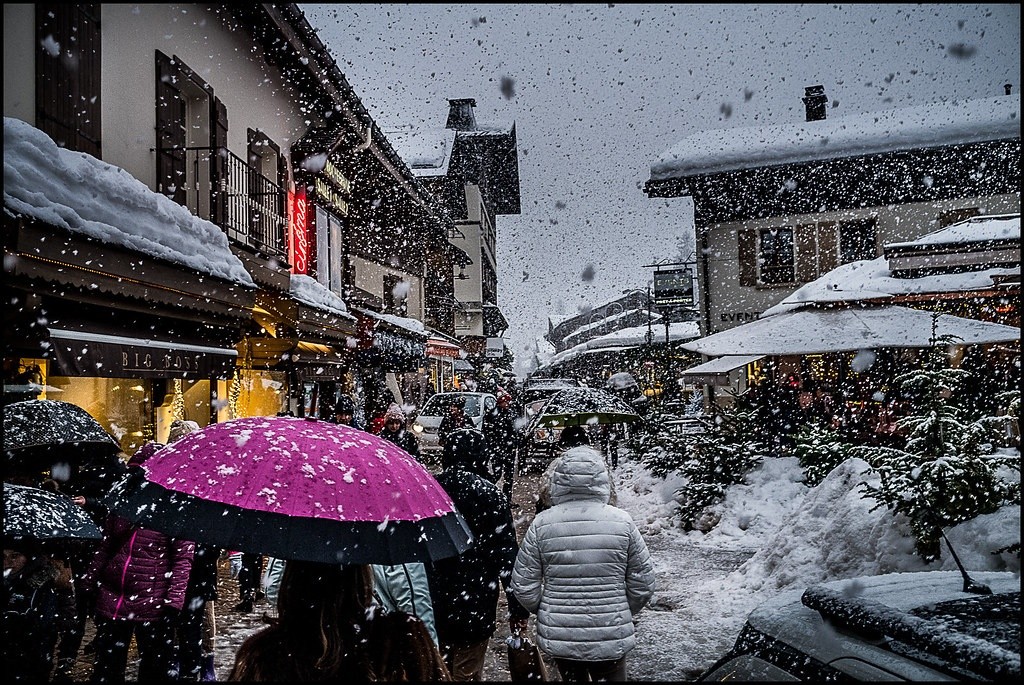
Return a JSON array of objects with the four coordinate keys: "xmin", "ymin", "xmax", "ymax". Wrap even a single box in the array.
[{"xmin": 506, "ymin": 635, "xmax": 546, "ymax": 684}]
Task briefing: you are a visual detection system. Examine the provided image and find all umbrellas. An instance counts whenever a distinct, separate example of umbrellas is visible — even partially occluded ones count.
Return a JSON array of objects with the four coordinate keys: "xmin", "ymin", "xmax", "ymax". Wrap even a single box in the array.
[
  {"xmin": 0, "ymin": 399, "xmax": 120, "ymax": 451},
  {"xmin": 101, "ymin": 416, "xmax": 473, "ymax": 562},
  {"xmin": 0, "ymin": 483, "xmax": 102, "ymax": 541}
]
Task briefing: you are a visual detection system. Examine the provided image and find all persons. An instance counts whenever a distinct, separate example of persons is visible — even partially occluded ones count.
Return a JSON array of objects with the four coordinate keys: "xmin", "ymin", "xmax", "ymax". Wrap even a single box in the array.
[{"xmin": 0, "ymin": 395, "xmax": 653, "ymax": 685}]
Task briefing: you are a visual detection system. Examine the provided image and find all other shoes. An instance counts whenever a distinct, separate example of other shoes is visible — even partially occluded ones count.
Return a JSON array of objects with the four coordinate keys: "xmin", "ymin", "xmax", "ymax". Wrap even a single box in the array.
[
  {"xmin": 252, "ymin": 591, "xmax": 265, "ymax": 602},
  {"xmin": 506, "ymin": 499, "xmax": 520, "ymax": 509},
  {"xmin": 84, "ymin": 637, "xmax": 98, "ymax": 654},
  {"xmin": 232, "ymin": 595, "xmax": 253, "ymax": 614}
]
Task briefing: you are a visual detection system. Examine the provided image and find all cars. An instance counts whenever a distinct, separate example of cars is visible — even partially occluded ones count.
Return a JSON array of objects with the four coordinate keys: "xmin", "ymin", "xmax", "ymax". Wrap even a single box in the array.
[
  {"xmin": 408, "ymin": 392, "xmax": 497, "ymax": 454},
  {"xmin": 694, "ymin": 572, "xmax": 1020, "ymax": 681}
]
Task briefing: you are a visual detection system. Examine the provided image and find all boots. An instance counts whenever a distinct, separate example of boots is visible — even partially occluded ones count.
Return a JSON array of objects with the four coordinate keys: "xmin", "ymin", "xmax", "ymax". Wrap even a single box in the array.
[
  {"xmin": 198, "ymin": 654, "xmax": 215, "ymax": 683},
  {"xmin": 165, "ymin": 661, "xmax": 179, "ymax": 682}
]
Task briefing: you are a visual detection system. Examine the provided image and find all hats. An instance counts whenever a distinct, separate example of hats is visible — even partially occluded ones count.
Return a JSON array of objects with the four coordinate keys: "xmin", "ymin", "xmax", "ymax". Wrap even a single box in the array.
[
  {"xmin": 335, "ymin": 393, "xmax": 355, "ymax": 415},
  {"xmin": 494, "ymin": 386, "xmax": 512, "ymax": 404},
  {"xmin": 442, "ymin": 428, "xmax": 491, "ymax": 468},
  {"xmin": 2, "ymin": 532, "xmax": 31, "ymax": 561},
  {"xmin": 451, "ymin": 396, "xmax": 466, "ymax": 409},
  {"xmin": 384, "ymin": 402, "xmax": 406, "ymax": 426},
  {"xmin": 168, "ymin": 419, "xmax": 199, "ymax": 443}
]
